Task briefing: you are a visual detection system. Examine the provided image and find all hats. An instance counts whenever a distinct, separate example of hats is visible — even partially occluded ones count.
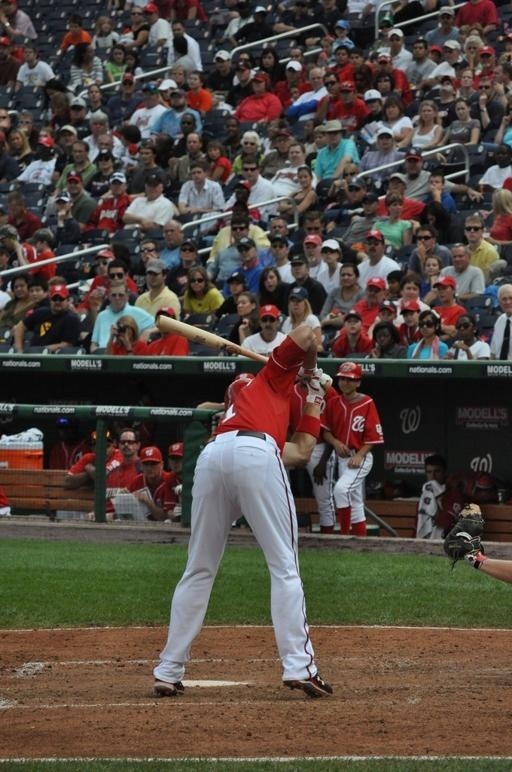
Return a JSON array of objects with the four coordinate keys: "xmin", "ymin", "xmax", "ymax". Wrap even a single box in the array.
[
  {"xmin": 168, "ymin": 441, "xmax": 184, "ymax": 457},
  {"xmin": 140, "ymin": 446, "xmax": 164, "ymax": 462},
  {"xmin": 336, "ymin": 361, "xmax": 363, "ymax": 380},
  {"xmin": 0, "ymin": 0, "xmax": 495, "ymax": 334}
]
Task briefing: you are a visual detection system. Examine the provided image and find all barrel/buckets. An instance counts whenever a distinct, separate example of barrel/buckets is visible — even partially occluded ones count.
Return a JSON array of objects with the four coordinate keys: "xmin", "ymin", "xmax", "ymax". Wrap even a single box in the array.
[{"xmin": 181, "ymin": 425, "xmax": 209, "ymax": 529}]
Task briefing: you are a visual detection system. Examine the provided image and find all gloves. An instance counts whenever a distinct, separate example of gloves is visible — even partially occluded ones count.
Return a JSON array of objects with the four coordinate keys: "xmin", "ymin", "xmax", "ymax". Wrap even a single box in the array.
[{"xmin": 297, "ymin": 361, "xmax": 334, "ymax": 403}]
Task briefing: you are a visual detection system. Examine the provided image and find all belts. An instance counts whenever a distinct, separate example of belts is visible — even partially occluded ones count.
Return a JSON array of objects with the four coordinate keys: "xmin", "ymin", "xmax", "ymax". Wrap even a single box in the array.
[{"xmin": 202, "ymin": 430, "xmax": 269, "ymax": 442}]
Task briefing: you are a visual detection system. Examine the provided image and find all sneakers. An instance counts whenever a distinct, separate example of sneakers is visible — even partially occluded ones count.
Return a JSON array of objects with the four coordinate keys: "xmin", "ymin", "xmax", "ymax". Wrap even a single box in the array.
[
  {"xmin": 149, "ymin": 677, "xmax": 188, "ymax": 696},
  {"xmin": 281, "ymin": 672, "xmax": 334, "ymax": 698}
]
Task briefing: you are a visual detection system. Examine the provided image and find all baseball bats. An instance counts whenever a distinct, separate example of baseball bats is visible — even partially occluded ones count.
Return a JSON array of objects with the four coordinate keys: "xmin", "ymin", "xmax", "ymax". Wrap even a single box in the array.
[{"xmin": 155, "ymin": 315, "xmax": 334, "ymax": 390}]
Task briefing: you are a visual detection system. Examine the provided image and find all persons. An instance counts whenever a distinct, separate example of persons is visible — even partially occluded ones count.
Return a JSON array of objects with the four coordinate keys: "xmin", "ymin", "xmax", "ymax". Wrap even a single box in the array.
[
  {"xmin": 466, "ymin": 551, "xmax": 512, "ymax": 584},
  {"xmin": 151, "ymin": 324, "xmax": 332, "ymax": 697}
]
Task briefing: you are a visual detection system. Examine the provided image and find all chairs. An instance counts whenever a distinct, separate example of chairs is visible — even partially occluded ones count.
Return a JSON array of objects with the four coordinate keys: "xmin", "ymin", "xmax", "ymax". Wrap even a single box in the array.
[{"xmin": 1, "ymin": 2, "xmax": 512, "ymax": 361}]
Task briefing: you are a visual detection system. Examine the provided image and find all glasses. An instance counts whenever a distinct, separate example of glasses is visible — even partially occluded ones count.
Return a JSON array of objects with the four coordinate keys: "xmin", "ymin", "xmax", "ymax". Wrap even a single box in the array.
[{"xmin": 118, "ymin": 439, "xmax": 140, "ymax": 445}]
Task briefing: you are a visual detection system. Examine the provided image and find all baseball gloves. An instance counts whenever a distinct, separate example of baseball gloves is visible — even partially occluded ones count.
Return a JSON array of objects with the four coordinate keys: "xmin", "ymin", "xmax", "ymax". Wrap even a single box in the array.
[{"xmin": 444, "ymin": 504, "xmax": 485, "ymax": 560}]
